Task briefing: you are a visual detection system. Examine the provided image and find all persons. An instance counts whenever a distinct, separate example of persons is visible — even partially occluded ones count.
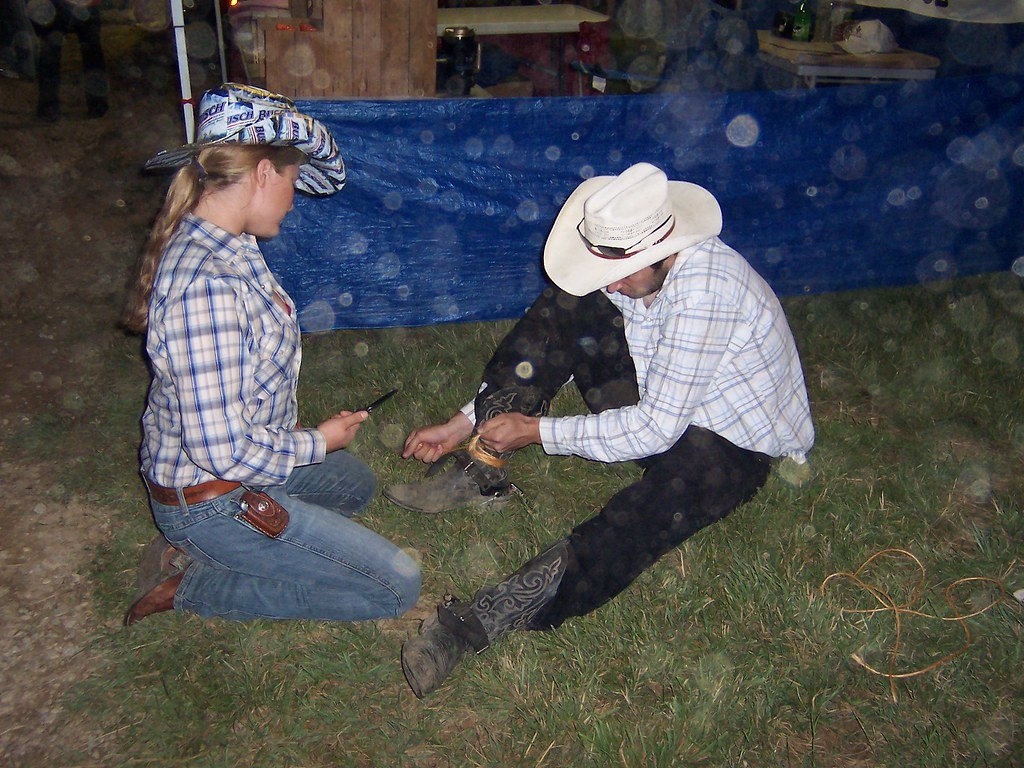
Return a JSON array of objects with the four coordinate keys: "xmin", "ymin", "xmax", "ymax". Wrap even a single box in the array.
[
  {"xmin": 122, "ymin": 81, "xmax": 423, "ymax": 629},
  {"xmin": 27, "ymin": 0, "xmax": 109, "ymax": 122},
  {"xmin": 380, "ymin": 161, "xmax": 817, "ymax": 700}
]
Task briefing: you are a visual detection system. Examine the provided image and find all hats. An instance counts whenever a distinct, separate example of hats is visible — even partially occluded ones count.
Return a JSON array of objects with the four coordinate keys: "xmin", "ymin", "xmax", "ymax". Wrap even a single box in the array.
[
  {"xmin": 542, "ymin": 162, "xmax": 722, "ymax": 297},
  {"xmin": 144, "ymin": 82, "xmax": 346, "ymax": 195},
  {"xmin": 832, "ymin": 20, "xmax": 898, "ymax": 55}
]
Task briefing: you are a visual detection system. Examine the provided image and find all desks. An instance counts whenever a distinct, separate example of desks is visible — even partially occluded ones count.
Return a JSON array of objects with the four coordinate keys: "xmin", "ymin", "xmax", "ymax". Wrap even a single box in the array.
[
  {"xmin": 757, "ymin": 30, "xmax": 941, "ymax": 90},
  {"xmin": 437, "ymin": 4, "xmax": 610, "ymax": 96}
]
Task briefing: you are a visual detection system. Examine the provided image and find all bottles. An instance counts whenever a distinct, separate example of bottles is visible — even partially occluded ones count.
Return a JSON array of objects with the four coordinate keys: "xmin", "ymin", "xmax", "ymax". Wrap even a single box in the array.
[
  {"xmin": 792, "ymin": 0, "xmax": 810, "ymax": 41},
  {"xmin": 816, "ymin": 0, "xmax": 830, "ymax": 42}
]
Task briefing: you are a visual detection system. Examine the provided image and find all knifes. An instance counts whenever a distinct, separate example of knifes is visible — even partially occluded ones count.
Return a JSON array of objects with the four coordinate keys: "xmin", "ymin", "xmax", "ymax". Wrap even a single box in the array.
[{"xmin": 353, "ymin": 389, "xmax": 398, "ymax": 413}]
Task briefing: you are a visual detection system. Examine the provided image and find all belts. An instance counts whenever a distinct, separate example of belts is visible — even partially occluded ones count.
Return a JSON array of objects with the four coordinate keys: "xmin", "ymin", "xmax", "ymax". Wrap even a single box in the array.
[{"xmin": 143, "ymin": 476, "xmax": 241, "ymax": 508}]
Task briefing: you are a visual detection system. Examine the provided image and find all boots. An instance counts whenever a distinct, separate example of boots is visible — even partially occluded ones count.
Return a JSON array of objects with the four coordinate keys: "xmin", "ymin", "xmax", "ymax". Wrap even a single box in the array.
[
  {"xmin": 382, "ymin": 382, "xmax": 552, "ymax": 513},
  {"xmin": 27, "ymin": 9, "xmax": 62, "ymax": 124},
  {"xmin": 400, "ymin": 538, "xmax": 568, "ymax": 699},
  {"xmin": 68, "ymin": 3, "xmax": 110, "ymax": 118}
]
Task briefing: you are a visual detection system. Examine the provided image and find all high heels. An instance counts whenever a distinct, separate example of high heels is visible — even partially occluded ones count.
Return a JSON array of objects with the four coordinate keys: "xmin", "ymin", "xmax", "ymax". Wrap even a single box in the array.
[{"xmin": 124, "ymin": 548, "xmax": 194, "ymax": 629}]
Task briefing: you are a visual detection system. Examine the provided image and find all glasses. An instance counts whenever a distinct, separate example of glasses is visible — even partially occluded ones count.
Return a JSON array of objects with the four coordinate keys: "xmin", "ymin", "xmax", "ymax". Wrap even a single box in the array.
[{"xmin": 576, "ymin": 215, "xmax": 673, "ymax": 258}]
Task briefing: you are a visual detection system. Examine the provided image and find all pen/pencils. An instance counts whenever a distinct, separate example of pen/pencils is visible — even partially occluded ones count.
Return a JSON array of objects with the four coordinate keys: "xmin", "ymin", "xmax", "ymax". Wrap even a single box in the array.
[{"xmin": 362, "ymin": 387, "xmax": 400, "ymax": 412}]
